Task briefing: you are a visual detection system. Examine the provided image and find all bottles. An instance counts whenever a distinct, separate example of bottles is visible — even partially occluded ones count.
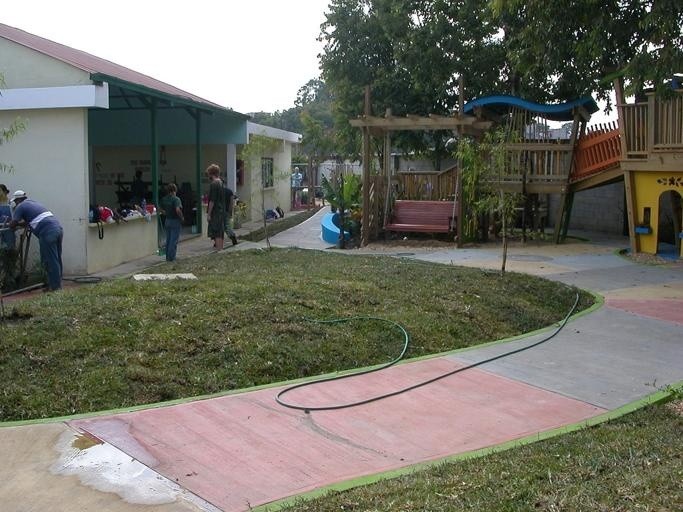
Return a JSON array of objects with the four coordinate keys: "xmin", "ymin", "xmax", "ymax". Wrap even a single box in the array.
[
  {"xmin": 141, "ymin": 199, "xmax": 147, "ymax": 216},
  {"xmin": 204, "ymin": 192, "xmax": 208, "ymax": 204}
]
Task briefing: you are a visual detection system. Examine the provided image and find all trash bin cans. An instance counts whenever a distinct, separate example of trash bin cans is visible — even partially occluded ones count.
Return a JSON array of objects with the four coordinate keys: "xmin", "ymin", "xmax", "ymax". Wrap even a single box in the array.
[
  {"xmin": 233, "ymin": 205, "xmax": 242, "ymax": 229},
  {"xmin": 191, "ymin": 208, "xmax": 198, "ymax": 234}
]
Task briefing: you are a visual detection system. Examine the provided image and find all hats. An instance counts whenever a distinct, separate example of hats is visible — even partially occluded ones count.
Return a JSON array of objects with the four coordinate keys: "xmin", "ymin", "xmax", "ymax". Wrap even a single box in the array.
[{"xmin": 8, "ymin": 189, "xmax": 26, "ymax": 204}]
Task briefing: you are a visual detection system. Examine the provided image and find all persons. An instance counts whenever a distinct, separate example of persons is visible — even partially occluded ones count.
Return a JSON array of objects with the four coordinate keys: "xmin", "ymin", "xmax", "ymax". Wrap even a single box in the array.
[
  {"xmin": 9, "ymin": 190, "xmax": 64, "ymax": 290},
  {"xmin": 206, "ymin": 164, "xmax": 226, "ymax": 252},
  {"xmin": 0, "ymin": 183, "xmax": 25, "ymax": 286},
  {"xmin": 291, "ymin": 166, "xmax": 303, "ymax": 207},
  {"xmin": 207, "ymin": 176, "xmax": 238, "ymax": 248},
  {"xmin": 160, "ymin": 183, "xmax": 185, "ymax": 263}
]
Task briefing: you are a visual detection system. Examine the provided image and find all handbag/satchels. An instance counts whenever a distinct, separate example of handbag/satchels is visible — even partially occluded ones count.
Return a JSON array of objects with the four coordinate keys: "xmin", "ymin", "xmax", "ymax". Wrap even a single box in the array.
[{"xmin": 90, "ymin": 207, "xmax": 100, "ymax": 222}]
